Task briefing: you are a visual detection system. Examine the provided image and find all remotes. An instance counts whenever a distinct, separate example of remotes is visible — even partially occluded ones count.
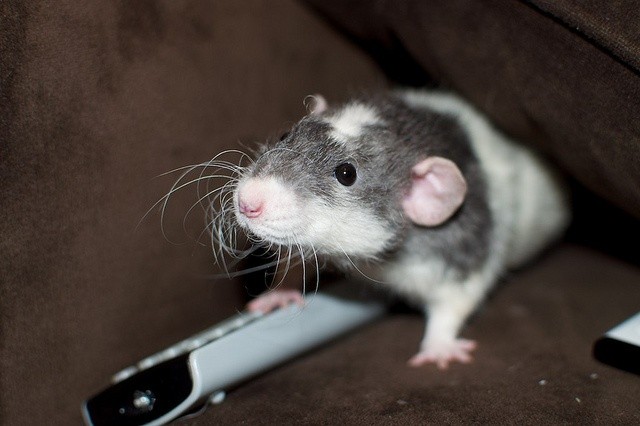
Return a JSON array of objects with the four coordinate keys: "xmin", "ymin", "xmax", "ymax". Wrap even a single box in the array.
[{"xmin": 81, "ymin": 269, "xmax": 395, "ymax": 425}]
